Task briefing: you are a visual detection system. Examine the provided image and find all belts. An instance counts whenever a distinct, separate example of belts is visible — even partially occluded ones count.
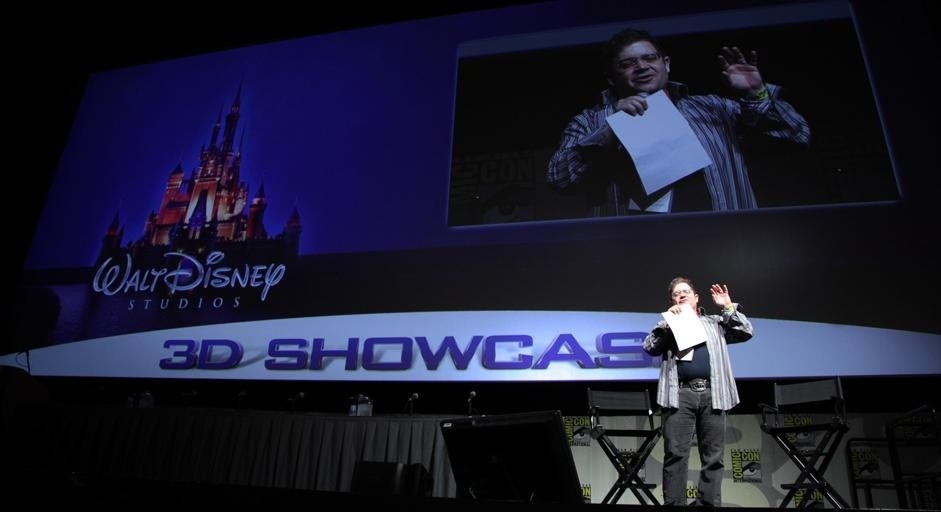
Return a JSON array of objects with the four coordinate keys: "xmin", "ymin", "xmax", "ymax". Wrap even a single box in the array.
[{"xmin": 677, "ymin": 378, "xmax": 711, "ymax": 393}]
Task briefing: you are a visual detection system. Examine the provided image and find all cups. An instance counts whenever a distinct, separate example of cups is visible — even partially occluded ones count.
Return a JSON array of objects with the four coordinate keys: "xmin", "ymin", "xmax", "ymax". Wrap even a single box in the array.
[{"xmin": 348, "ymin": 404, "xmax": 356, "ymax": 417}]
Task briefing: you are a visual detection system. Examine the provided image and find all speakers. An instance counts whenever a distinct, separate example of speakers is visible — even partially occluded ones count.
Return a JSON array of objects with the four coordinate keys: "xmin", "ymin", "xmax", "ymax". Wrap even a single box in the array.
[{"xmin": 350, "ymin": 460, "xmax": 432, "ymax": 497}]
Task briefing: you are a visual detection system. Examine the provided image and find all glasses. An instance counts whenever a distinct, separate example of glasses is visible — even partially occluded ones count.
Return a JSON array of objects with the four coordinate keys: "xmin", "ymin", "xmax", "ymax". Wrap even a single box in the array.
[
  {"xmin": 617, "ymin": 54, "xmax": 663, "ymax": 70},
  {"xmin": 672, "ymin": 289, "xmax": 693, "ymax": 296}
]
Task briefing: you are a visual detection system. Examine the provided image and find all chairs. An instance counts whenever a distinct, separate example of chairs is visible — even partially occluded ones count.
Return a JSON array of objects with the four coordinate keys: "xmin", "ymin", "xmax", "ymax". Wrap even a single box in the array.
[
  {"xmin": 587, "ymin": 385, "xmax": 664, "ymax": 512},
  {"xmin": 757, "ymin": 374, "xmax": 854, "ymax": 510}
]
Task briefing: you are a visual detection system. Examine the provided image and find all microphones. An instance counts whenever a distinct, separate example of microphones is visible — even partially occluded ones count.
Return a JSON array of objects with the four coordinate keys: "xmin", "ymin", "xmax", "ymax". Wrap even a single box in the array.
[
  {"xmin": 288, "ymin": 392, "xmax": 304, "ymax": 401},
  {"xmin": 409, "ymin": 392, "xmax": 418, "ymax": 401},
  {"xmin": 466, "ymin": 391, "xmax": 477, "ymax": 403}
]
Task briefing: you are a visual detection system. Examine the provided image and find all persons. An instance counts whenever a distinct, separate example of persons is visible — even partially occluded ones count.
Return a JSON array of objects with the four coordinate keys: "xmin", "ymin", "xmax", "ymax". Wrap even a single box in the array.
[
  {"xmin": 641, "ymin": 274, "xmax": 756, "ymax": 508},
  {"xmin": 543, "ymin": 24, "xmax": 815, "ymax": 213}
]
